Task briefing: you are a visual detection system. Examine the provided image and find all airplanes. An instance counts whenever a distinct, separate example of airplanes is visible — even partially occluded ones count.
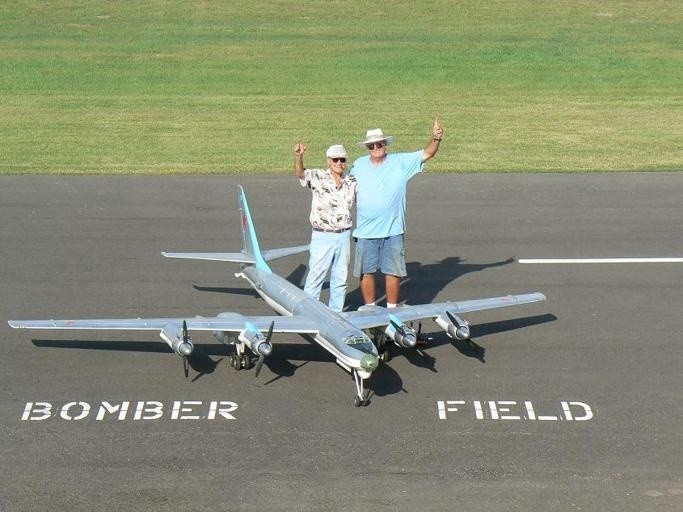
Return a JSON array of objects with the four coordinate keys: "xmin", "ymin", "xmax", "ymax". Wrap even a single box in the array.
[{"xmin": 4, "ymin": 184, "xmax": 546, "ymax": 409}]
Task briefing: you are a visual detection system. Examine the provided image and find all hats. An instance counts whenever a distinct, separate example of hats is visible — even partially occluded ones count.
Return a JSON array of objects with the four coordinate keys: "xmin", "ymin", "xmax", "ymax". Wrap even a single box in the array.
[
  {"xmin": 356, "ymin": 128, "xmax": 394, "ymax": 150},
  {"xmin": 326, "ymin": 145, "xmax": 348, "ymax": 159}
]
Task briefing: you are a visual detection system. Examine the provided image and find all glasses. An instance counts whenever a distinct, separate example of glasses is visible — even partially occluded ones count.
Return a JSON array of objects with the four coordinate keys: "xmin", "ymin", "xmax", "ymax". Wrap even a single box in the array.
[
  {"xmin": 332, "ymin": 158, "xmax": 346, "ymax": 163},
  {"xmin": 368, "ymin": 143, "xmax": 382, "ymax": 150}
]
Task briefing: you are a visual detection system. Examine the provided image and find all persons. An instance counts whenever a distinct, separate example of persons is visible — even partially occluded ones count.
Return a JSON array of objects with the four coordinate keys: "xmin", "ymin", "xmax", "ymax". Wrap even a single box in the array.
[
  {"xmin": 346, "ymin": 117, "xmax": 444, "ymax": 309},
  {"xmin": 294, "ymin": 143, "xmax": 359, "ymax": 314}
]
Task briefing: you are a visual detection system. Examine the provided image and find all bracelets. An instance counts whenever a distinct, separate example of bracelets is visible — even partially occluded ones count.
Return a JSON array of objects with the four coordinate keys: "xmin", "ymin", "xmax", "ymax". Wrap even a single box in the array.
[{"xmin": 434, "ymin": 137, "xmax": 442, "ymax": 142}]
